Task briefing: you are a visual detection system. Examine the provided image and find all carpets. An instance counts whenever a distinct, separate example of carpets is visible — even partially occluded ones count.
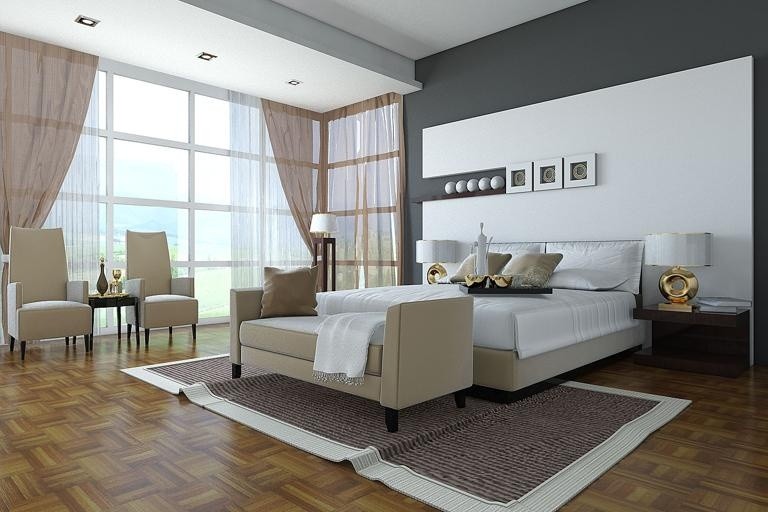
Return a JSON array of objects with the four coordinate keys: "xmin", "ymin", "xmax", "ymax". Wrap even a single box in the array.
[{"xmin": 120, "ymin": 350, "xmax": 694, "ymax": 512}]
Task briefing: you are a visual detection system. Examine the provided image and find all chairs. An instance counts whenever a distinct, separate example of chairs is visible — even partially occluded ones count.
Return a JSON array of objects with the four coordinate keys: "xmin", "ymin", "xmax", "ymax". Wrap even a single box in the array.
[
  {"xmin": 126, "ymin": 228, "xmax": 198, "ymax": 351},
  {"xmin": 7, "ymin": 226, "xmax": 92, "ymax": 361}
]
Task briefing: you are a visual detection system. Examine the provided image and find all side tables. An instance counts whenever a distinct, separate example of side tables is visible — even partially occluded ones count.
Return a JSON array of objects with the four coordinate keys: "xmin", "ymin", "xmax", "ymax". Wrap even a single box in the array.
[{"xmin": 89, "ymin": 294, "xmax": 141, "ymax": 352}]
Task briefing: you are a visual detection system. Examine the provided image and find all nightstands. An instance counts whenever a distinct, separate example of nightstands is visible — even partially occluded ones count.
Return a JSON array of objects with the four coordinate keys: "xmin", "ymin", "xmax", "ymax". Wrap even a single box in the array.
[{"xmin": 632, "ymin": 303, "xmax": 750, "ymax": 377}]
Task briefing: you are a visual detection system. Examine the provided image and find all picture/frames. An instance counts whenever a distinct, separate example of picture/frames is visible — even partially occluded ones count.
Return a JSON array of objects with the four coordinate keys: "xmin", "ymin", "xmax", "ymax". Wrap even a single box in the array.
[{"xmin": 506, "ymin": 153, "xmax": 597, "ymax": 194}]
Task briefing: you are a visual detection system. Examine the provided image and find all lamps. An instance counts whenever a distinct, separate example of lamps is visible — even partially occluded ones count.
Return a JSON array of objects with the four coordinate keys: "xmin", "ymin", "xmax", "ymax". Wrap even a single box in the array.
[
  {"xmin": 416, "ymin": 240, "xmax": 456, "ymax": 285},
  {"xmin": 644, "ymin": 232, "xmax": 713, "ymax": 310},
  {"xmin": 310, "ymin": 212, "xmax": 338, "ymax": 238}
]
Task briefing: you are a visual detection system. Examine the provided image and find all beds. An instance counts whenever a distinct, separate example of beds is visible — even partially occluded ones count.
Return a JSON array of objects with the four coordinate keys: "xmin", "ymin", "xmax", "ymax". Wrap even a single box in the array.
[{"xmin": 318, "ymin": 240, "xmax": 650, "ymax": 403}]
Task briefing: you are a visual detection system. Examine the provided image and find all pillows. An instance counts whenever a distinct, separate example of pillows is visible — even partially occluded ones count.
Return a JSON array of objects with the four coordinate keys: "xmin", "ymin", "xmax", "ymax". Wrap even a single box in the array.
[
  {"xmin": 499, "ymin": 253, "xmax": 564, "ymax": 288},
  {"xmin": 450, "ymin": 252, "xmax": 511, "ymax": 284},
  {"xmin": 261, "ymin": 265, "xmax": 318, "ymax": 316}
]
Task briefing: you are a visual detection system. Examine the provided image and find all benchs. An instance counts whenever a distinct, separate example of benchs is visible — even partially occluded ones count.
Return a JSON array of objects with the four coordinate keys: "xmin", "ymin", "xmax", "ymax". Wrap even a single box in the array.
[{"xmin": 229, "ymin": 288, "xmax": 475, "ymax": 431}]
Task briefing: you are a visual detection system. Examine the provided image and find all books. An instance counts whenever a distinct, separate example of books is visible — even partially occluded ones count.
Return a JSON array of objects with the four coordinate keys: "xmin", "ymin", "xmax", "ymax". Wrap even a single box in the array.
[
  {"xmin": 696, "ymin": 297, "xmax": 752, "ymax": 306},
  {"xmin": 700, "ymin": 305, "xmax": 738, "ymax": 313}
]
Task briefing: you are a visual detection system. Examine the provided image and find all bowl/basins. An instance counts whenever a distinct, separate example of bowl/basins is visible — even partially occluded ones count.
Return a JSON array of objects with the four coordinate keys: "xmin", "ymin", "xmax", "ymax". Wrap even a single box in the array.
[
  {"xmin": 464, "ymin": 274, "xmax": 488, "ymax": 288},
  {"xmin": 489, "ymin": 275, "xmax": 512, "ymax": 288}
]
